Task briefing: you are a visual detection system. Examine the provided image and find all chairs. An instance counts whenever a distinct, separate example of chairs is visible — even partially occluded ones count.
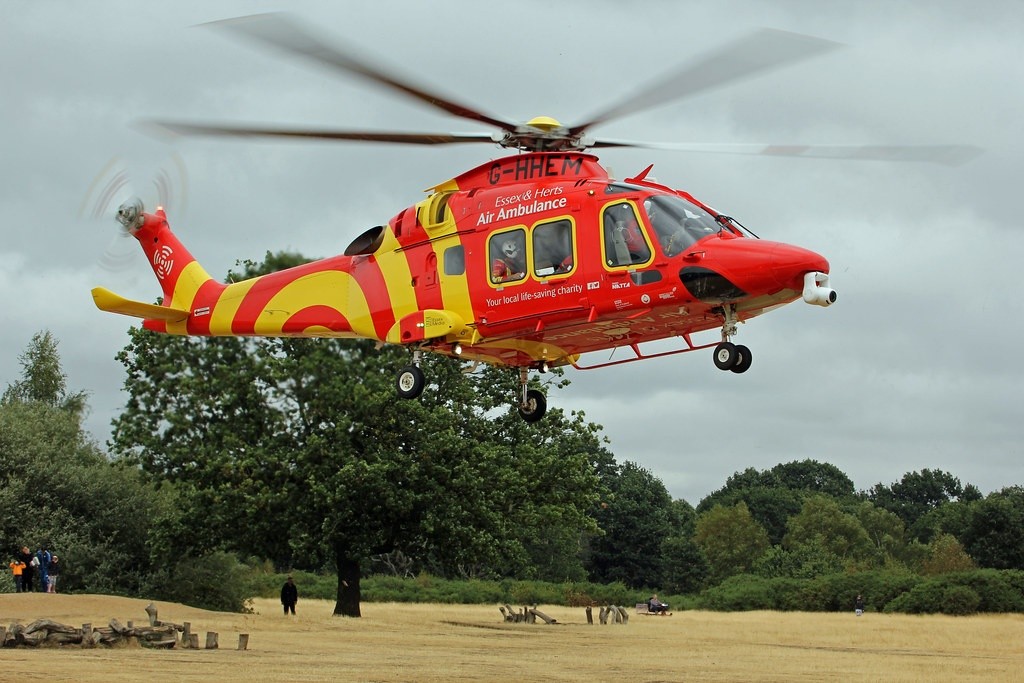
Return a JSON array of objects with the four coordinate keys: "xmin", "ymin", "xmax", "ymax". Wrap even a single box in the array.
[
  {"xmin": 647, "ymin": 598, "xmax": 660, "ymax": 615},
  {"xmin": 613, "ymin": 221, "xmax": 632, "ymax": 264}
]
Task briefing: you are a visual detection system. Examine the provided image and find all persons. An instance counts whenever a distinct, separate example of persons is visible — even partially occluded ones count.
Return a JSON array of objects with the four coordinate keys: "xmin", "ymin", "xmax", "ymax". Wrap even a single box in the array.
[
  {"xmin": 557, "ymin": 256, "xmax": 573, "ymax": 273},
  {"xmin": 491, "ymin": 241, "xmax": 523, "ymax": 283},
  {"xmin": 624, "ymin": 204, "xmax": 659, "ymax": 259},
  {"xmin": 281, "ymin": 576, "xmax": 297, "ymax": 615},
  {"xmin": 9, "ymin": 545, "xmax": 58, "ymax": 593},
  {"xmin": 648, "ymin": 594, "xmax": 669, "ymax": 615}
]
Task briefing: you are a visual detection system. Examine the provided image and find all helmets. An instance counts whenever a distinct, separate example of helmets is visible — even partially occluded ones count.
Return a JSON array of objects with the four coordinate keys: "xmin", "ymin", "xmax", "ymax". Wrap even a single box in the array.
[{"xmin": 502, "ymin": 240, "xmax": 522, "ymax": 259}]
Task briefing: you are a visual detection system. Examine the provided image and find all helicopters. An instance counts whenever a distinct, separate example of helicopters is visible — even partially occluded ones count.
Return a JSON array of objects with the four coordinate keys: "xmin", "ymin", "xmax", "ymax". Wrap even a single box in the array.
[{"xmin": 78, "ymin": 7, "xmax": 991, "ymax": 426}]
